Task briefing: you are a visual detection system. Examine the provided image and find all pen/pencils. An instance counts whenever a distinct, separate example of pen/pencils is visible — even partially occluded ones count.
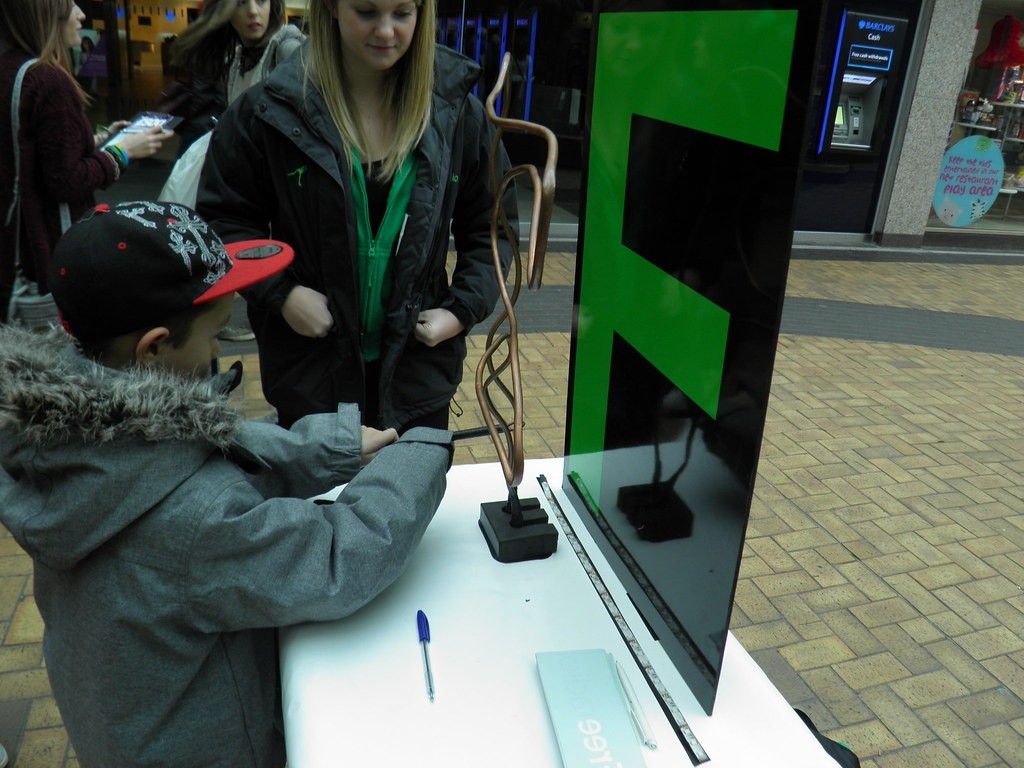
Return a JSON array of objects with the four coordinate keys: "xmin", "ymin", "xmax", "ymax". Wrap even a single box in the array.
[
  {"xmin": 614, "ymin": 657, "xmax": 659, "ymax": 750},
  {"xmin": 416, "ymin": 609, "xmax": 437, "ymax": 705}
]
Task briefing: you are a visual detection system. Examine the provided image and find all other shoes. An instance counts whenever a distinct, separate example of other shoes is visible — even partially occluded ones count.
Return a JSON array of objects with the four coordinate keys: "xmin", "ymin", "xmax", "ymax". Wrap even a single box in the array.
[
  {"xmin": 221, "ymin": 360, "xmax": 242, "ymax": 397},
  {"xmin": 218, "ymin": 325, "xmax": 256, "ymax": 342}
]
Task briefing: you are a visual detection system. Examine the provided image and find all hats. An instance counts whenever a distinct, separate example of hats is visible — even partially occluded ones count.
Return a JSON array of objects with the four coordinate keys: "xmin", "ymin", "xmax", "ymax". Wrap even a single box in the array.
[{"xmin": 50, "ymin": 200, "xmax": 294, "ymax": 348}]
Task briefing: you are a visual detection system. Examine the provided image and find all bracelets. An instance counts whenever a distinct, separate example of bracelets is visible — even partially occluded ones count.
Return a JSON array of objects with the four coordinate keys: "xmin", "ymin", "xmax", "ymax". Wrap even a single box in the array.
[
  {"xmin": 96, "ymin": 125, "xmax": 114, "ymax": 142},
  {"xmin": 106, "ymin": 145, "xmax": 128, "ymax": 167}
]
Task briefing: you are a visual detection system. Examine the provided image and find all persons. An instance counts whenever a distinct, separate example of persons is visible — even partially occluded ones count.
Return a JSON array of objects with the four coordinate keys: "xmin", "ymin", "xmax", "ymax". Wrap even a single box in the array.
[
  {"xmin": 169, "ymin": 0, "xmax": 307, "ymax": 211},
  {"xmin": 0, "ymin": 201, "xmax": 456, "ymax": 768},
  {"xmin": 197, "ymin": 0, "xmax": 520, "ymax": 431},
  {"xmin": 0, "ymin": 0, "xmax": 175, "ymax": 325}
]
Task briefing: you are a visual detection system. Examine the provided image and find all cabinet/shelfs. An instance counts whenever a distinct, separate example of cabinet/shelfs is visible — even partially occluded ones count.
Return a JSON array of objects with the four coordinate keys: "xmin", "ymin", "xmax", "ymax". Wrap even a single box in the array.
[{"xmin": 948, "ymin": 65, "xmax": 1024, "ymax": 222}]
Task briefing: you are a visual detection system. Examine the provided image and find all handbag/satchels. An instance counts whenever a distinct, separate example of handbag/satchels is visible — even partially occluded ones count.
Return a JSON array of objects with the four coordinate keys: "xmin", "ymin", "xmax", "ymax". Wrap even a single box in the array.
[
  {"xmin": 7, "ymin": 274, "xmax": 65, "ymax": 334},
  {"xmin": 158, "ymin": 130, "xmax": 213, "ymax": 210}
]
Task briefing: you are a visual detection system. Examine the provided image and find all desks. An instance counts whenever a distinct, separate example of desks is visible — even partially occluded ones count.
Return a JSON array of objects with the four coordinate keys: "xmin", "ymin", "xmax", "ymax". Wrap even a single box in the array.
[{"xmin": 277, "ymin": 458, "xmax": 844, "ymax": 768}]
